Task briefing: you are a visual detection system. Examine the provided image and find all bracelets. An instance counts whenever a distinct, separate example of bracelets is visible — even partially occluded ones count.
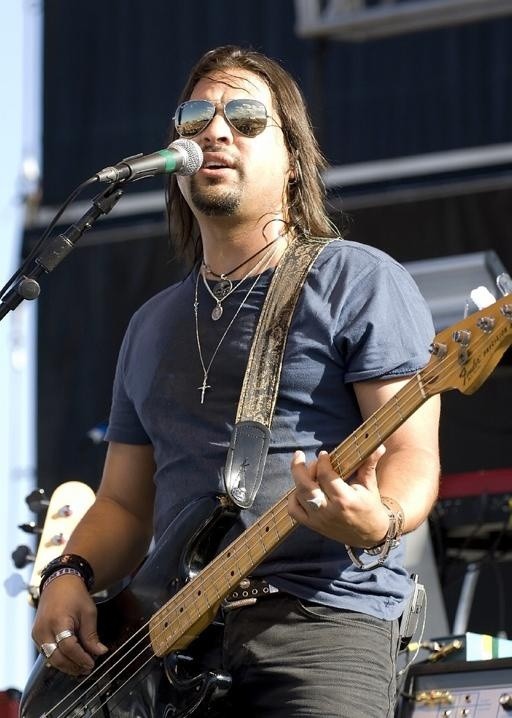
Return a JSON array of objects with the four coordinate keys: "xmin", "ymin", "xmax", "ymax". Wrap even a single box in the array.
[
  {"xmin": 39, "ymin": 554, "xmax": 96, "ymax": 592},
  {"xmin": 345, "ymin": 510, "xmax": 396, "ymax": 571}
]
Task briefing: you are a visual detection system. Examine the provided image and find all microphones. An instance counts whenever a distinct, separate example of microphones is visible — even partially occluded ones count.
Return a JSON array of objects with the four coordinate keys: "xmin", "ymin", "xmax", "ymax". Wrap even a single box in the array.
[{"xmin": 96, "ymin": 138, "xmax": 205, "ymax": 182}]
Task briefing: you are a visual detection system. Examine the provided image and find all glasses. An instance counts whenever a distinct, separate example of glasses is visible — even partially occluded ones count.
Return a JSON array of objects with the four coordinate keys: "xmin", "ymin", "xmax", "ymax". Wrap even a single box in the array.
[{"xmin": 171, "ymin": 99, "xmax": 284, "ymax": 141}]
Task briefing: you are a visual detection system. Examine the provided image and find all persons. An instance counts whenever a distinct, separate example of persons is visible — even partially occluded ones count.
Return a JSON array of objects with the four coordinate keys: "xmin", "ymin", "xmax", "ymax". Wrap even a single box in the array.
[{"xmin": 31, "ymin": 48, "xmax": 441, "ymax": 718}]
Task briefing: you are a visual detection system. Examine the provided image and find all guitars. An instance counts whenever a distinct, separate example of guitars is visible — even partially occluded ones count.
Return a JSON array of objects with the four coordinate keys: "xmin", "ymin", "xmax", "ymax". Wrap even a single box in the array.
[{"xmin": 18, "ymin": 272, "xmax": 512, "ymax": 718}]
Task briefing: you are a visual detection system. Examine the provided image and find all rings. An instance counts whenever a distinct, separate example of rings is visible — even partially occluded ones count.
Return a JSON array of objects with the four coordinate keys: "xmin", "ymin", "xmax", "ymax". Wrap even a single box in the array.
[
  {"xmin": 307, "ymin": 494, "xmax": 325, "ymax": 511},
  {"xmin": 41, "ymin": 641, "xmax": 57, "ymax": 670},
  {"xmin": 56, "ymin": 628, "xmax": 75, "ymax": 646}
]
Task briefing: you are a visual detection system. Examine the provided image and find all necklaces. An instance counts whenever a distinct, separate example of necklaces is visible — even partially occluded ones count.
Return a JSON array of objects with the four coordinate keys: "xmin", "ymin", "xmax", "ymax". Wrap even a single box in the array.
[{"xmin": 194, "ymin": 232, "xmax": 289, "ymax": 404}]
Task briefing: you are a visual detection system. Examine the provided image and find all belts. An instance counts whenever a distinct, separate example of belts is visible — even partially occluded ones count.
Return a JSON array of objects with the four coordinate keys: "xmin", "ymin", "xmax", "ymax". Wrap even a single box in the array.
[{"xmin": 164, "ymin": 579, "xmax": 280, "ymax": 609}]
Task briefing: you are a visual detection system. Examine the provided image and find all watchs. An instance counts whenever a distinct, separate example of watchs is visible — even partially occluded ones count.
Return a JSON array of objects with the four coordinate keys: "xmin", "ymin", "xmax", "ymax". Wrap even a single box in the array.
[{"xmin": 364, "ymin": 497, "xmax": 404, "ymax": 556}]
